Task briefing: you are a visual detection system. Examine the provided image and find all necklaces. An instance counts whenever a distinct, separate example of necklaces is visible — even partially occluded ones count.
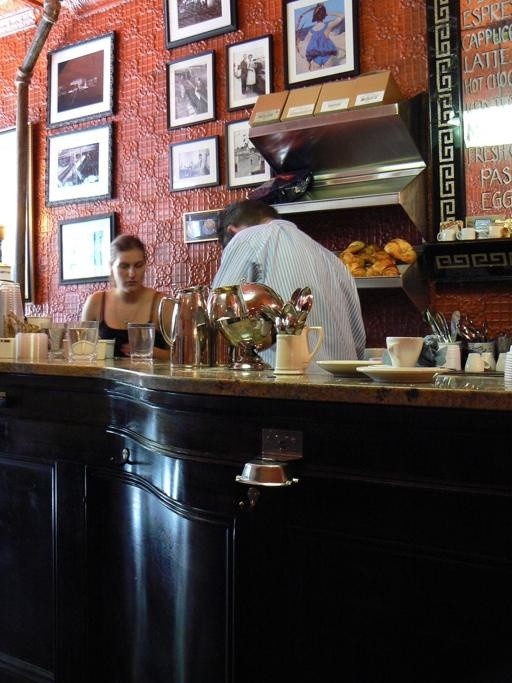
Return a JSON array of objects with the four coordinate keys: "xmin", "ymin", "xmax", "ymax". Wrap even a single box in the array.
[{"xmin": 119, "ymin": 301, "xmax": 144, "ymax": 323}]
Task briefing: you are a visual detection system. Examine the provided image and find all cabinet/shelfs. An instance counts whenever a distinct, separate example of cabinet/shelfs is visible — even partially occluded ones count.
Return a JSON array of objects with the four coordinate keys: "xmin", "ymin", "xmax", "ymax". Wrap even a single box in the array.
[{"xmin": 248, "ymin": 87, "xmax": 438, "ymax": 325}]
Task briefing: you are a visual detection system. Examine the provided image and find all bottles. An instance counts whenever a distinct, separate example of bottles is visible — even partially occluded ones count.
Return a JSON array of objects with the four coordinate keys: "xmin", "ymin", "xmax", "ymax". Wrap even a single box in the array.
[{"xmin": 488, "ymin": 222, "xmax": 505, "ymax": 239}]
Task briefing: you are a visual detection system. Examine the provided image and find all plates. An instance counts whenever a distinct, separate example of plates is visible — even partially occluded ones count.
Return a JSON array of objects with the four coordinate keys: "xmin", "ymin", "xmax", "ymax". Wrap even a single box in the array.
[
  {"xmin": 502, "ymin": 351, "xmax": 512, "ymax": 381},
  {"xmin": 358, "ymin": 364, "xmax": 448, "ymax": 385},
  {"xmin": 315, "ymin": 360, "xmax": 382, "ymax": 379}
]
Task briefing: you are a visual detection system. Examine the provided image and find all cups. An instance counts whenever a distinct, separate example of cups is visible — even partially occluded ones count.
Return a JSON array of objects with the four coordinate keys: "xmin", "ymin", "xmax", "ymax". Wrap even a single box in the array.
[
  {"xmin": 275, "ymin": 324, "xmax": 325, "ymax": 377},
  {"xmin": 66, "ymin": 318, "xmax": 99, "ymax": 363},
  {"xmin": 127, "ymin": 321, "xmax": 155, "ymax": 362},
  {"xmin": 464, "ymin": 352, "xmax": 490, "ymax": 373},
  {"xmin": 474, "ymin": 218, "xmax": 491, "ymax": 239},
  {"xmin": 435, "ymin": 227, "xmax": 477, "ymax": 241},
  {"xmin": 436, "ymin": 340, "xmax": 462, "ymax": 371},
  {"xmin": 385, "ymin": 336, "xmax": 426, "ymax": 367},
  {"xmin": 494, "ymin": 353, "xmax": 507, "ymax": 372},
  {"xmin": 48, "ymin": 322, "xmax": 68, "ymax": 361},
  {"xmin": 480, "ymin": 351, "xmax": 496, "ymax": 371}
]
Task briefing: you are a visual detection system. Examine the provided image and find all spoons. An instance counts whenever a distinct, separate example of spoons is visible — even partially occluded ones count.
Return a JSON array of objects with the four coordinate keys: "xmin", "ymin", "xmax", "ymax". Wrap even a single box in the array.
[{"xmin": 276, "ymin": 286, "xmax": 313, "ymax": 334}]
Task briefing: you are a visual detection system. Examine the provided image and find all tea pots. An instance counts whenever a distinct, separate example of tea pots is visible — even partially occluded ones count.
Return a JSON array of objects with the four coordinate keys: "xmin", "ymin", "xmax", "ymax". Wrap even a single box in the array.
[{"xmin": 158, "ymin": 281, "xmax": 214, "ymax": 367}]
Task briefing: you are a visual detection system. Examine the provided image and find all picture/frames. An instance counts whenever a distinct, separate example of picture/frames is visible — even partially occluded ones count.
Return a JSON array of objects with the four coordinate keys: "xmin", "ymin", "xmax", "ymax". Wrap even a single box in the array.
[
  {"xmin": 57, "ymin": 210, "xmax": 116, "ymax": 285},
  {"xmin": 44, "ymin": 120, "xmax": 112, "ymax": 208},
  {"xmin": 281, "ymin": 0, "xmax": 361, "ymax": 87},
  {"xmin": 0, "ymin": 121, "xmax": 34, "ymax": 305},
  {"xmin": 45, "ymin": 28, "xmax": 117, "ymax": 130},
  {"xmin": 163, "ymin": 1, "xmax": 274, "ymax": 245}
]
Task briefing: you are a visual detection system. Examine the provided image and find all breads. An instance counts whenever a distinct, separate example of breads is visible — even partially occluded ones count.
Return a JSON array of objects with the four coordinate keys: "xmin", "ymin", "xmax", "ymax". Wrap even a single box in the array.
[{"xmin": 339, "ymin": 238, "xmax": 417, "ymax": 277}]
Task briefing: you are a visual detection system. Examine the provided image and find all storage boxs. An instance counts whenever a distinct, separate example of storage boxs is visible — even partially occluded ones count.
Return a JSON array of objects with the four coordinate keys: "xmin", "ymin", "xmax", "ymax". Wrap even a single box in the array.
[
  {"xmin": 247, "ymin": 90, "xmax": 290, "ymax": 127},
  {"xmin": 280, "ymin": 82, "xmax": 322, "ymax": 120},
  {"xmin": 313, "ymin": 70, "xmax": 409, "ymax": 117}
]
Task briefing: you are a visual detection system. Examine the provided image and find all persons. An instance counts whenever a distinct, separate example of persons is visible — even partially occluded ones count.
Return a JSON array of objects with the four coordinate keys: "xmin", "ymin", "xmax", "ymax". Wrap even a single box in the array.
[
  {"xmin": 206, "ymin": 198, "xmax": 368, "ymax": 374},
  {"xmin": 240, "ymin": 52, "xmax": 248, "ymax": 95},
  {"xmin": 302, "ymin": 3, "xmax": 344, "ymax": 71},
  {"xmin": 81, "ymin": 233, "xmax": 174, "ymax": 359},
  {"xmin": 198, "ymin": 153, "xmax": 205, "ymax": 175},
  {"xmin": 246, "ymin": 54, "xmax": 258, "ymax": 93},
  {"xmin": 177, "ymin": 66, "xmax": 204, "ymax": 108}
]
